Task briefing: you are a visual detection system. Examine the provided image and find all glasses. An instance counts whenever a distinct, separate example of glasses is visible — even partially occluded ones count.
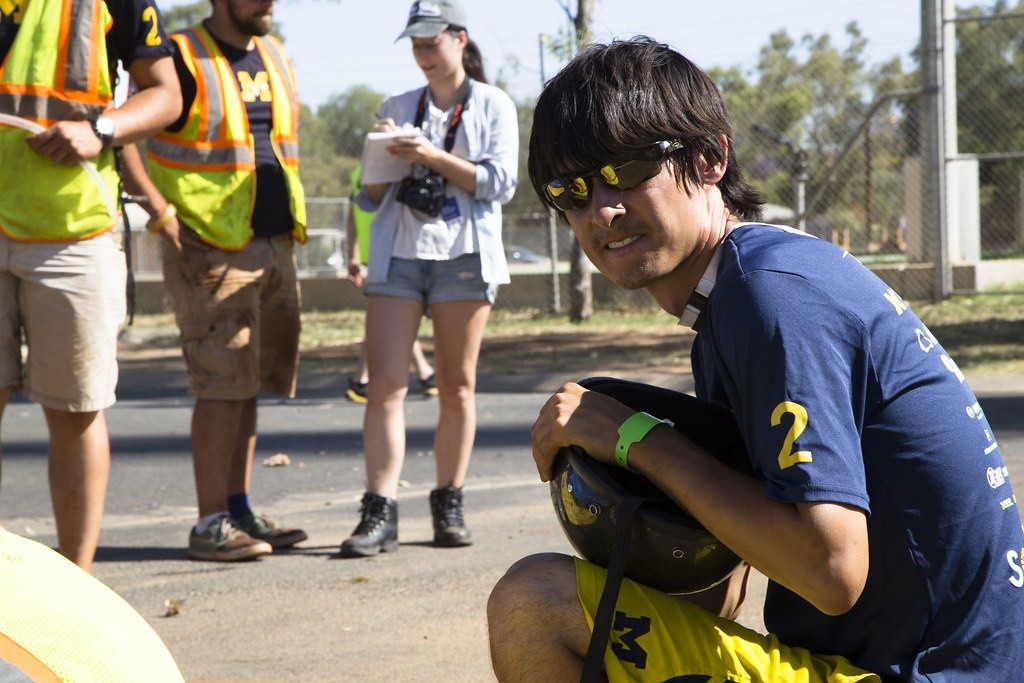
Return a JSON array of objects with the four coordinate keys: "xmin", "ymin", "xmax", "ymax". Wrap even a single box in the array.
[{"xmin": 542, "ymin": 139, "xmax": 689, "ymax": 210}]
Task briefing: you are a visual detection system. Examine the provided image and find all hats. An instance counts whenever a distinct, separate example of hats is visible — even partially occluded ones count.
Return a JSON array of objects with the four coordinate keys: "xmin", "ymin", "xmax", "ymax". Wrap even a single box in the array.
[{"xmin": 393, "ymin": 0, "xmax": 467, "ymax": 42}]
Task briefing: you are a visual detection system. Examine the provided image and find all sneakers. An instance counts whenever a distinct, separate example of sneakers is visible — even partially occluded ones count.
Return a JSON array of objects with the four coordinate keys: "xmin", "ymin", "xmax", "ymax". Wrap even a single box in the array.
[
  {"xmin": 429, "ymin": 481, "xmax": 472, "ymax": 546},
  {"xmin": 341, "ymin": 491, "xmax": 399, "ymax": 554},
  {"xmin": 237, "ymin": 513, "xmax": 306, "ymax": 546},
  {"xmin": 189, "ymin": 516, "xmax": 272, "ymax": 559}
]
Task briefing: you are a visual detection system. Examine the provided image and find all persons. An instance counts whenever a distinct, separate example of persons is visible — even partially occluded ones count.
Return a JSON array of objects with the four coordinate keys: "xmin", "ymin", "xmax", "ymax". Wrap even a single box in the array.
[
  {"xmin": 116, "ymin": 0, "xmax": 309, "ymax": 562},
  {"xmin": 341, "ymin": 0, "xmax": 520, "ymax": 554},
  {"xmin": 488, "ymin": 42, "xmax": 1024, "ymax": 683},
  {"xmin": 0, "ymin": 0, "xmax": 184, "ymax": 573}
]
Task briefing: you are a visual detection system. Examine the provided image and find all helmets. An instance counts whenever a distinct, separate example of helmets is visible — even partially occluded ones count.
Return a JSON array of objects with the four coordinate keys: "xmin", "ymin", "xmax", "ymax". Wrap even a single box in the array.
[{"xmin": 549, "ymin": 377, "xmax": 766, "ymax": 595}]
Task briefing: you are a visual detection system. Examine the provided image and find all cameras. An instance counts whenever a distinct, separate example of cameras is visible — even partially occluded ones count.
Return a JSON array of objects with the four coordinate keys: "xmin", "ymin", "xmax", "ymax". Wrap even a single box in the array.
[{"xmin": 395, "ymin": 176, "xmax": 446, "ymax": 216}]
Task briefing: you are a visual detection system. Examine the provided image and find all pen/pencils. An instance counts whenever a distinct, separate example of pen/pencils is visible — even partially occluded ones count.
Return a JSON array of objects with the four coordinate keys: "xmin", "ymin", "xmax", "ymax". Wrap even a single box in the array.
[{"xmin": 373, "ymin": 113, "xmax": 387, "ymax": 126}]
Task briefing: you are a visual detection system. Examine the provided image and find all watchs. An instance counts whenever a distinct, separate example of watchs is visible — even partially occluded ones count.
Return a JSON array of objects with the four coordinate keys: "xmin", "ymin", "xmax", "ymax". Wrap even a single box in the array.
[{"xmin": 89, "ymin": 114, "xmax": 114, "ymax": 151}]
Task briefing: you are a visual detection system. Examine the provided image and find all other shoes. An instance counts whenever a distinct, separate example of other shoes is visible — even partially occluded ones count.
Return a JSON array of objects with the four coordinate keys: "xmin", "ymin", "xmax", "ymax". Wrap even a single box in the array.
[
  {"xmin": 419, "ymin": 371, "xmax": 439, "ymax": 398},
  {"xmin": 345, "ymin": 378, "xmax": 369, "ymax": 403}
]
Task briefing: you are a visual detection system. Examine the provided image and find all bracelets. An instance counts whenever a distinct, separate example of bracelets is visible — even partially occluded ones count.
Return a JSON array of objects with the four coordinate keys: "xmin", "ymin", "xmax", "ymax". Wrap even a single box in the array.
[
  {"xmin": 145, "ymin": 202, "xmax": 178, "ymax": 233},
  {"xmin": 614, "ymin": 412, "xmax": 669, "ymax": 471}
]
